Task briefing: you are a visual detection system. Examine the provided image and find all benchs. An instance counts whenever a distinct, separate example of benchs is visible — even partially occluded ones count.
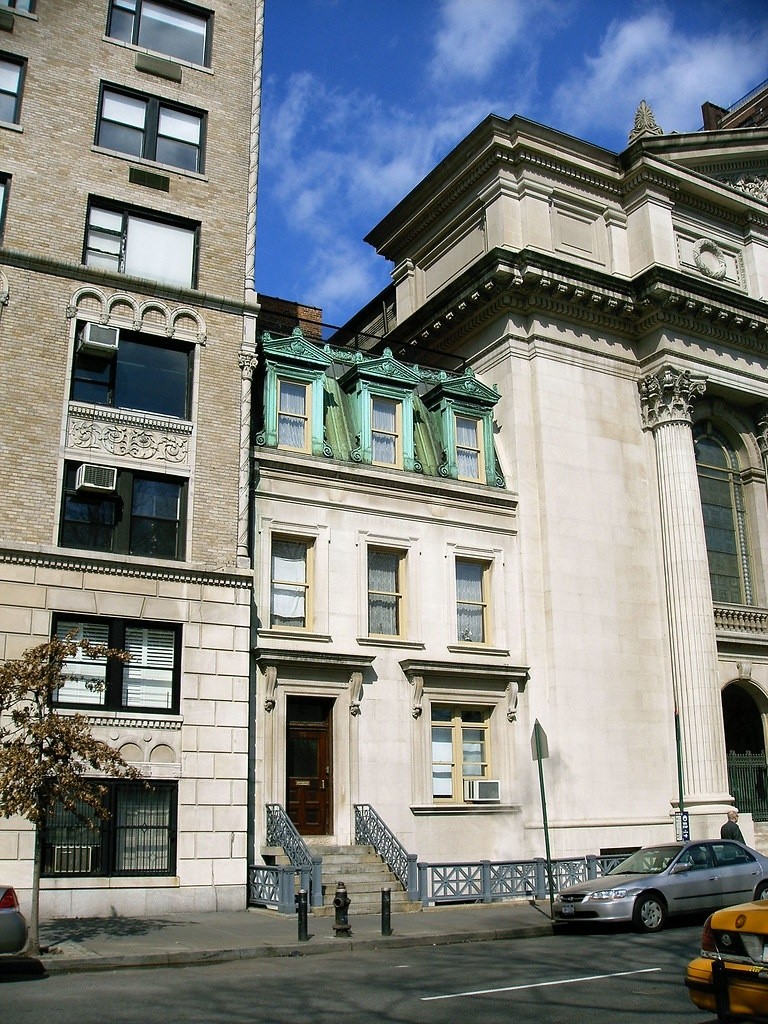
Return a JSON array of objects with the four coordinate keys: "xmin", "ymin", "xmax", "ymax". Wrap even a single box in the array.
[{"xmin": 718, "ymin": 856, "xmax": 749, "ymax": 867}]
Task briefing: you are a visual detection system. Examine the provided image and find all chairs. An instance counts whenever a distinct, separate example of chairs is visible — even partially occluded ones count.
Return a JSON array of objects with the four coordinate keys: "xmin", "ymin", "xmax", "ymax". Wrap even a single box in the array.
[{"xmin": 692, "ymin": 852, "xmax": 710, "ymax": 871}]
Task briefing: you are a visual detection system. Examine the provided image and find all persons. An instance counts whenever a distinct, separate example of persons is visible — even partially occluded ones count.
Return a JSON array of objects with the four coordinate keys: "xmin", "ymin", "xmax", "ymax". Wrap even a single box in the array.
[{"xmin": 721, "ymin": 811, "xmax": 746, "ymax": 858}]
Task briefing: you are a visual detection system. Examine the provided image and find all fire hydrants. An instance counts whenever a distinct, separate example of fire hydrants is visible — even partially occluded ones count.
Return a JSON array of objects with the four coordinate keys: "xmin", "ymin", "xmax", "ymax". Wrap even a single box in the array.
[{"xmin": 332, "ymin": 884, "xmax": 352, "ymax": 936}]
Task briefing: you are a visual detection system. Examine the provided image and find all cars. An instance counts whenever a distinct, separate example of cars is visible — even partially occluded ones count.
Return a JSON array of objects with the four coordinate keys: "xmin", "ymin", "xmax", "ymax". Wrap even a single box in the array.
[
  {"xmin": 549, "ymin": 838, "xmax": 768, "ymax": 932},
  {"xmin": 685, "ymin": 896, "xmax": 767, "ymax": 1022},
  {"xmin": 0, "ymin": 885, "xmax": 29, "ymax": 956}
]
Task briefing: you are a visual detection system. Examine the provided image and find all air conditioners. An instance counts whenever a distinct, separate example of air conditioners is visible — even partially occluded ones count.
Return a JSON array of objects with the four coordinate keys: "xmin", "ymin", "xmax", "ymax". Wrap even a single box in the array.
[
  {"xmin": 77, "ymin": 322, "xmax": 119, "ymax": 358},
  {"xmin": 464, "ymin": 779, "xmax": 500, "ymax": 801},
  {"xmin": 51, "ymin": 844, "xmax": 91, "ymax": 873},
  {"xmin": 75, "ymin": 464, "xmax": 117, "ymax": 495}
]
